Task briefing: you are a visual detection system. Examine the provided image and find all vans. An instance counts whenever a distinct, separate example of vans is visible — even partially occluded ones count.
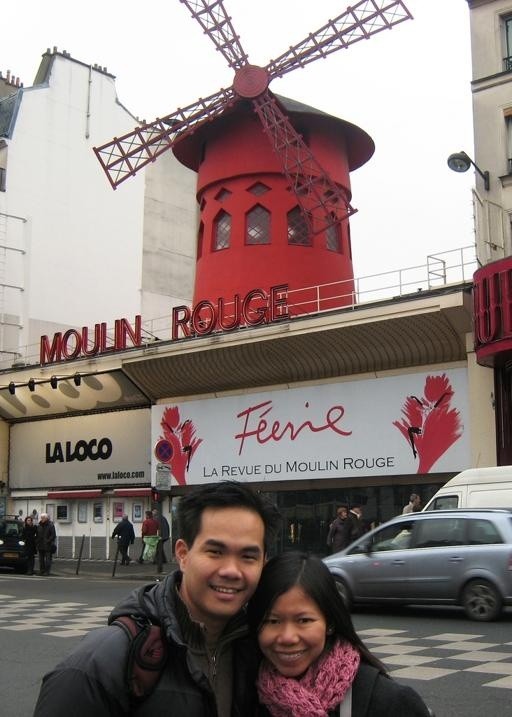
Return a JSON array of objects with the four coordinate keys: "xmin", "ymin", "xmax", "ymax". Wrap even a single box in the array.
[{"xmin": 392, "ymin": 467, "xmax": 512, "ymax": 544}]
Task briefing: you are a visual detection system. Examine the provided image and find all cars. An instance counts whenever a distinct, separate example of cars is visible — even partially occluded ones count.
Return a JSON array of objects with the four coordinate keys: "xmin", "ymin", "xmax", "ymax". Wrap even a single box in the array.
[
  {"xmin": 321, "ymin": 508, "xmax": 512, "ymax": 622},
  {"xmin": 0, "ymin": 514, "xmax": 35, "ymax": 574}
]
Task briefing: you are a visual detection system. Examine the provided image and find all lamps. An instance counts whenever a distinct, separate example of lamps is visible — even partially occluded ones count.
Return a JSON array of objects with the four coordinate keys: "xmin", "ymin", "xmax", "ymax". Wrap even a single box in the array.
[{"xmin": 448, "ymin": 151, "xmax": 488, "ymax": 190}]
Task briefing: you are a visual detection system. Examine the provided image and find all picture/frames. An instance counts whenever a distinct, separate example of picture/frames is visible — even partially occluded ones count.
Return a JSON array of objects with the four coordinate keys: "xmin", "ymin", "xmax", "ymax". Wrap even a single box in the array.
[{"xmin": 46, "ymin": 502, "xmax": 143, "ymax": 523}]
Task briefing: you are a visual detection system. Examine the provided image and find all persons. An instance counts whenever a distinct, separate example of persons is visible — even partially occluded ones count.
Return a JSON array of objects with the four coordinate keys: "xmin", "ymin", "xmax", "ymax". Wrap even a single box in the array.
[
  {"xmin": 30, "ymin": 477, "xmax": 288, "ymax": 717},
  {"xmin": 17, "ymin": 515, "xmax": 38, "ymax": 575},
  {"xmin": 13, "ymin": 509, "xmax": 24, "ymax": 521},
  {"xmin": 342, "ymin": 503, "xmax": 364, "ymax": 547},
  {"xmin": 402, "ymin": 493, "xmax": 423, "ymax": 515},
  {"xmin": 326, "ymin": 506, "xmax": 347, "ymax": 554},
  {"xmin": 238, "ymin": 548, "xmax": 434, "ymax": 717},
  {"xmin": 111, "ymin": 515, "xmax": 135, "ymax": 566},
  {"xmin": 151, "ymin": 507, "xmax": 170, "ymax": 564},
  {"xmin": 30, "ymin": 509, "xmax": 40, "ymax": 524},
  {"xmin": 388, "ymin": 522, "xmax": 411, "ymax": 548},
  {"xmin": 33, "ymin": 513, "xmax": 56, "ymax": 576},
  {"xmin": 137, "ymin": 510, "xmax": 162, "ymax": 565}
]
